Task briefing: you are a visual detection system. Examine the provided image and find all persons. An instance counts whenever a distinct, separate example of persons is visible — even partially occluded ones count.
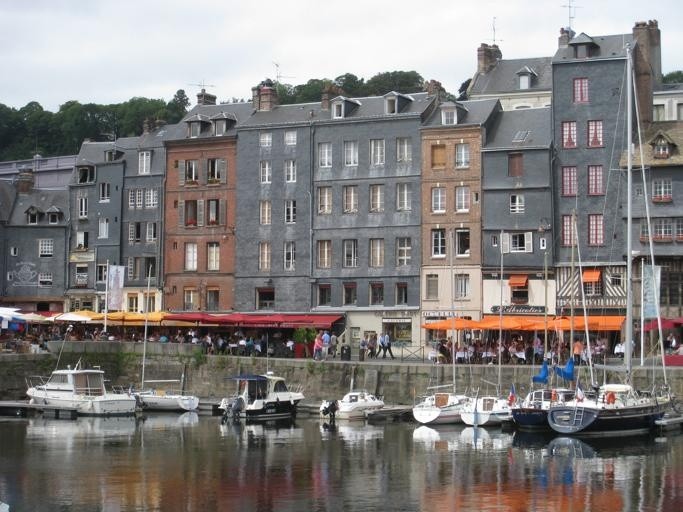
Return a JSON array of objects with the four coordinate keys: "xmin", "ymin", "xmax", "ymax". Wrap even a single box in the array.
[{"xmin": 1, "ymin": 324, "xmax": 677, "ymax": 361}]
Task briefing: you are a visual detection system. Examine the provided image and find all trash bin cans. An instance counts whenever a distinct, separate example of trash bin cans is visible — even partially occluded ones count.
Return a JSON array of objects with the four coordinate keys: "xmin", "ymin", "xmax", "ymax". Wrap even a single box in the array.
[{"xmin": 341, "ymin": 345, "xmax": 351, "ymax": 361}]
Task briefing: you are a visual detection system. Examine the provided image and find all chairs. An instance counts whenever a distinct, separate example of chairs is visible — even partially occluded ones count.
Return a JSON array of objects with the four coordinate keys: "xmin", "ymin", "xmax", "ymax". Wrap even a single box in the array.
[
  {"xmin": 204, "ymin": 338, "xmax": 293, "ymax": 359},
  {"xmin": 428, "ymin": 341, "xmax": 607, "ymax": 367}
]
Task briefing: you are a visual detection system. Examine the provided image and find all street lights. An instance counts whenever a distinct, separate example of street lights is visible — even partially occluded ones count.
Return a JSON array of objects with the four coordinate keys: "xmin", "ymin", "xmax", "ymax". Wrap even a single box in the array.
[{"xmin": 623, "ymin": 250, "xmax": 640, "ymax": 368}]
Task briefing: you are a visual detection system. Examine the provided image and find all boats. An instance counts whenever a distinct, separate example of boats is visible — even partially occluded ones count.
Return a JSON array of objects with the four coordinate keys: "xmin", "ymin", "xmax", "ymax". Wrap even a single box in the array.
[
  {"xmin": 24, "ymin": 323, "xmax": 137, "ymax": 414},
  {"xmin": 318, "ymin": 389, "xmax": 385, "ymax": 420},
  {"xmin": 213, "ymin": 334, "xmax": 304, "ymax": 419}
]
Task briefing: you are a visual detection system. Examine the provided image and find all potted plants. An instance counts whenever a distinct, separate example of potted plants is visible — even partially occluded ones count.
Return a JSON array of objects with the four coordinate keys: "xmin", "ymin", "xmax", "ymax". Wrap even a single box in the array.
[
  {"xmin": 291, "ymin": 327, "xmax": 307, "ymax": 358},
  {"xmin": 304, "ymin": 327, "xmax": 318, "ymax": 356}
]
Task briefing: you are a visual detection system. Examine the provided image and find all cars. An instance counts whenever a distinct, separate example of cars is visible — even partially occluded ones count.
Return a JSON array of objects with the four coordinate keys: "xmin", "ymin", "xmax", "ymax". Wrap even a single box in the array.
[{"xmin": 656, "ymin": 343, "xmax": 682, "ymax": 366}]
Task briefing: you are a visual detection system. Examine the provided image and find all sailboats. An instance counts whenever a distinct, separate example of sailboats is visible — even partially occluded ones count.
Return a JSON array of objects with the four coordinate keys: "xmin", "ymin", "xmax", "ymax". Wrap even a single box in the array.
[
  {"xmin": 546, "ymin": 42, "xmax": 676, "ymax": 436},
  {"xmin": 110, "ymin": 263, "xmax": 199, "ymax": 413},
  {"xmin": 510, "ymin": 208, "xmax": 599, "ymax": 426},
  {"xmin": 411, "ymin": 231, "xmax": 483, "ymax": 425},
  {"xmin": 459, "ymin": 228, "xmax": 520, "ymax": 429}
]
